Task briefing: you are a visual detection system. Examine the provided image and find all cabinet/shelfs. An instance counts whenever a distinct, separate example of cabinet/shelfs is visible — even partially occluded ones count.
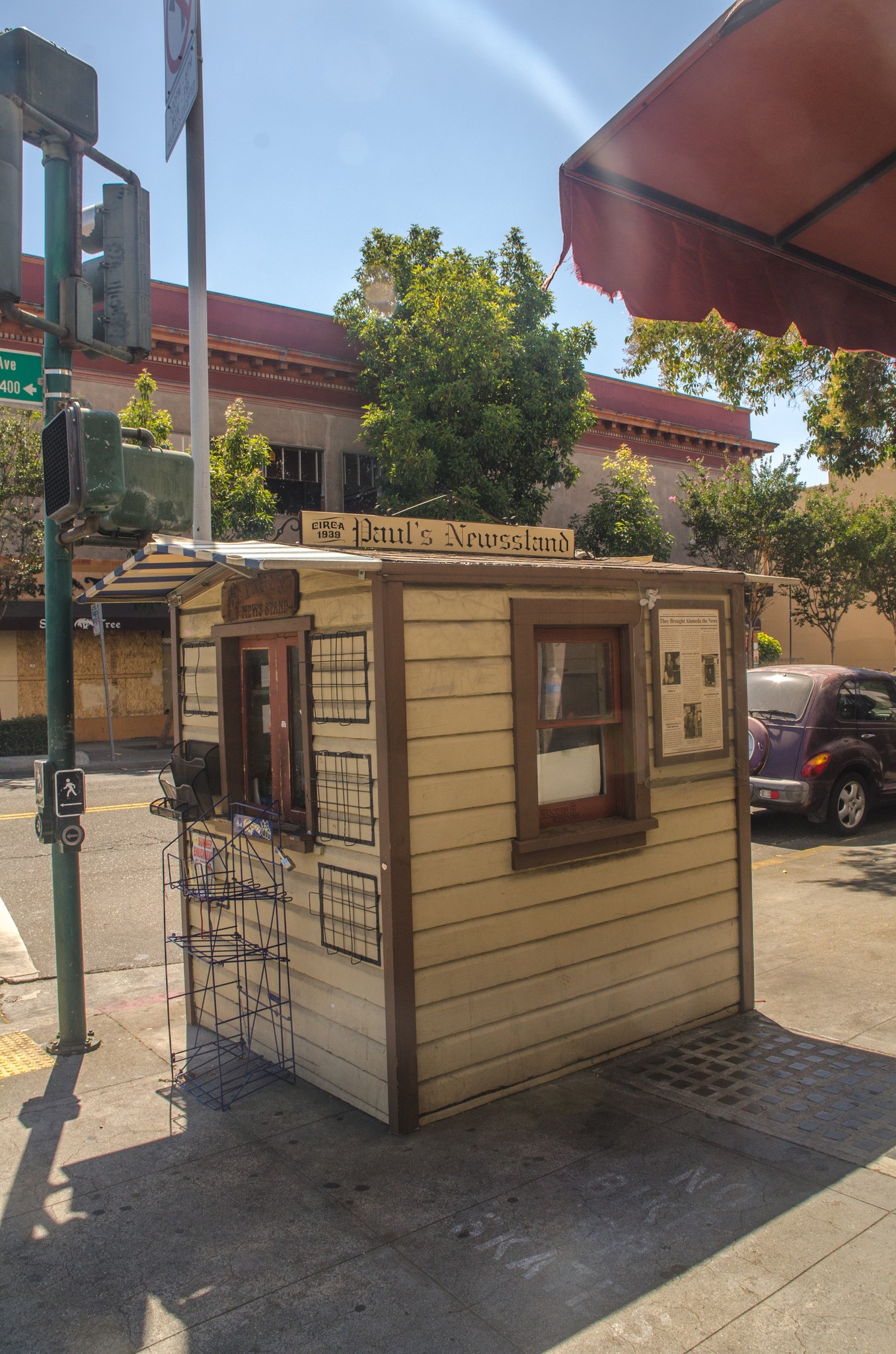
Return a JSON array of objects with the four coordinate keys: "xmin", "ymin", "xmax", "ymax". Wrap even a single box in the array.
[{"xmin": 161, "ymin": 793, "xmax": 295, "ymax": 1112}]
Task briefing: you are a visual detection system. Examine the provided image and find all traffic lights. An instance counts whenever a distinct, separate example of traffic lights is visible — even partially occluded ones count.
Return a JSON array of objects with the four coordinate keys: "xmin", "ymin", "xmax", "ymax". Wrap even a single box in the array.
[
  {"xmin": 41, "ymin": 397, "xmax": 126, "ymax": 526},
  {"xmin": 81, "ymin": 182, "xmax": 153, "ymax": 362}
]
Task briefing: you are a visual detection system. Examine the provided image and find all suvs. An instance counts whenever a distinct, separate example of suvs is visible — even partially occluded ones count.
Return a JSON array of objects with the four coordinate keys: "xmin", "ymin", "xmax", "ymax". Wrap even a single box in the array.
[{"xmin": 747, "ymin": 664, "xmax": 896, "ymax": 837}]
